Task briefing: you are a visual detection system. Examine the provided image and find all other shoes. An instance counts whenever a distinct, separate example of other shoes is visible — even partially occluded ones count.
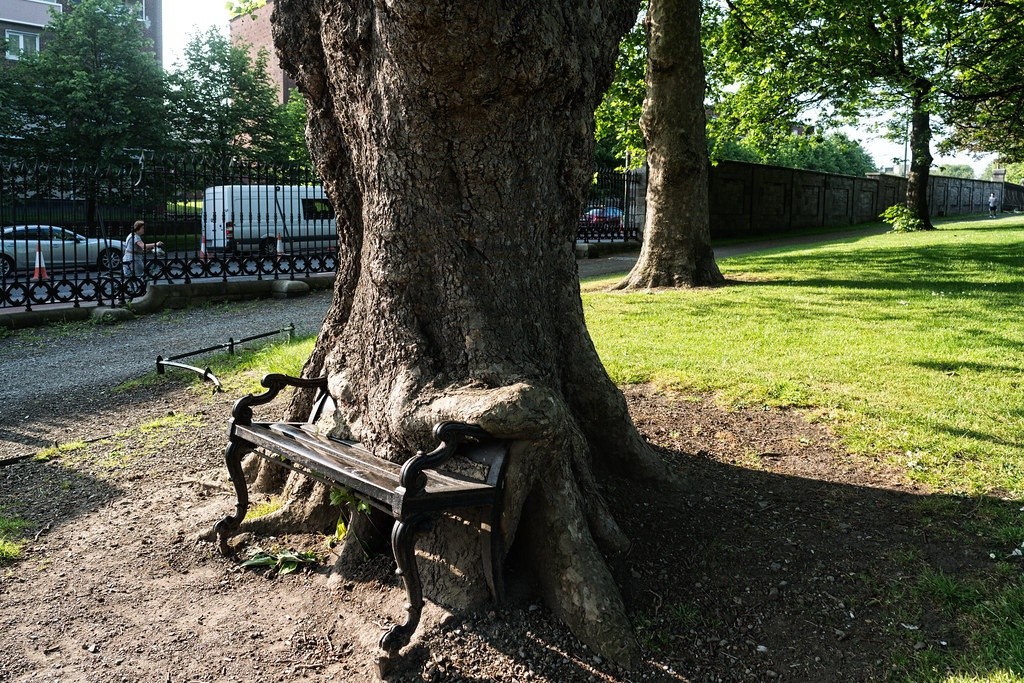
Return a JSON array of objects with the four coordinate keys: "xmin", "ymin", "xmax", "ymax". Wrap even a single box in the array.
[
  {"xmin": 994, "ymin": 216, "xmax": 996, "ymax": 219},
  {"xmin": 990, "ymin": 216, "xmax": 993, "ymax": 219}
]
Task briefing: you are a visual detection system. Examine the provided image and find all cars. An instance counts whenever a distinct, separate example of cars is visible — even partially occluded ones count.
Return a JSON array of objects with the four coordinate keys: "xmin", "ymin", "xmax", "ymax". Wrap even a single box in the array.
[
  {"xmin": 0, "ymin": 225, "xmax": 127, "ymax": 279},
  {"xmin": 580, "ymin": 206, "xmax": 623, "ymax": 232}
]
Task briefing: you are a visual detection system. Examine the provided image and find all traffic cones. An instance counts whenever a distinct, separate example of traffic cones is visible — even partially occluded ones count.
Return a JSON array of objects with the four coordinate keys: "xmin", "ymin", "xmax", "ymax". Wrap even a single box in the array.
[
  {"xmin": 30, "ymin": 244, "xmax": 51, "ymax": 281},
  {"xmin": 276, "ymin": 233, "xmax": 284, "ymax": 259},
  {"xmin": 196, "ymin": 235, "xmax": 215, "ymax": 266}
]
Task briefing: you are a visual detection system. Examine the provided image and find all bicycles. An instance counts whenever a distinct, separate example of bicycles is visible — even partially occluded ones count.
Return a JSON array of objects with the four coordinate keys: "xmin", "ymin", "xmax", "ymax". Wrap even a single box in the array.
[{"xmin": 96, "ymin": 242, "xmax": 182, "ymax": 300}]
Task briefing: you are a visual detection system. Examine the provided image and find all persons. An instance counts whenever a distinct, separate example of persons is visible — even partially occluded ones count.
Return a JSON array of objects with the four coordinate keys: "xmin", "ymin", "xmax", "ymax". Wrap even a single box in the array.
[
  {"xmin": 988, "ymin": 193, "xmax": 997, "ymax": 218},
  {"xmin": 121, "ymin": 220, "xmax": 164, "ymax": 299}
]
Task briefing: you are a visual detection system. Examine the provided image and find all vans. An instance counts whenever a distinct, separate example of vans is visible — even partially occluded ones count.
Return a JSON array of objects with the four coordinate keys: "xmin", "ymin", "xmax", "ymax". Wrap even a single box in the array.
[{"xmin": 202, "ymin": 185, "xmax": 340, "ymax": 258}]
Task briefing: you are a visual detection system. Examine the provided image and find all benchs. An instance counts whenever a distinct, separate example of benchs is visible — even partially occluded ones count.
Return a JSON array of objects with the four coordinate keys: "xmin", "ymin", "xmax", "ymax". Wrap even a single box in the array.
[{"xmin": 212, "ymin": 373, "xmax": 512, "ymax": 654}]
